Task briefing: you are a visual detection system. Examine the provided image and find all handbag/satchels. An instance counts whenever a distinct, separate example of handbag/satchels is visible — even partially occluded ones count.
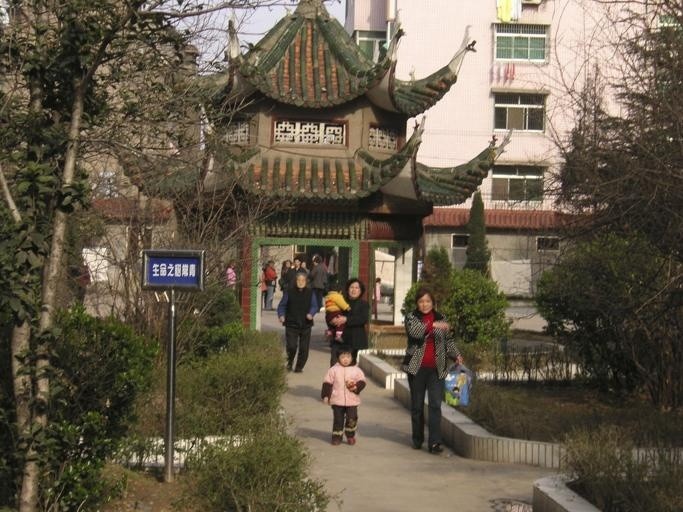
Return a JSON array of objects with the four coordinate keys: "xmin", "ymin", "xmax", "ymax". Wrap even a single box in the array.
[{"xmin": 445, "ymin": 362, "xmax": 473, "ymax": 407}]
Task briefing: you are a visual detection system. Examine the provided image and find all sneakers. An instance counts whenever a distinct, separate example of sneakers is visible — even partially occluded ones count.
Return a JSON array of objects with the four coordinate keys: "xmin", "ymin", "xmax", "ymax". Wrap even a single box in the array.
[{"xmin": 429, "ymin": 445, "xmax": 443, "ymax": 452}]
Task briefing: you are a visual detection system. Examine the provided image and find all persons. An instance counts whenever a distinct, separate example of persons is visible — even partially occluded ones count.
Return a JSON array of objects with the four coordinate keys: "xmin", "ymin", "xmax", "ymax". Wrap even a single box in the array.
[
  {"xmin": 321, "ymin": 345, "xmax": 365, "ymax": 445},
  {"xmin": 323, "ymin": 280, "xmax": 353, "ymax": 344},
  {"xmin": 224, "ymin": 248, "xmax": 381, "ymax": 319},
  {"xmin": 328, "ymin": 278, "xmax": 370, "ymax": 367},
  {"xmin": 405, "ymin": 288, "xmax": 463, "ymax": 453},
  {"xmin": 277, "ymin": 273, "xmax": 319, "ymax": 374}
]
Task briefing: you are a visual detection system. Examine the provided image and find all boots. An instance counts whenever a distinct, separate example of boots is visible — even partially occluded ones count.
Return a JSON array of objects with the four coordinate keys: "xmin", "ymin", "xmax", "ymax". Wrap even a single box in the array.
[
  {"xmin": 344, "ymin": 426, "xmax": 357, "ymax": 445},
  {"xmin": 331, "ymin": 428, "xmax": 345, "ymax": 444}
]
